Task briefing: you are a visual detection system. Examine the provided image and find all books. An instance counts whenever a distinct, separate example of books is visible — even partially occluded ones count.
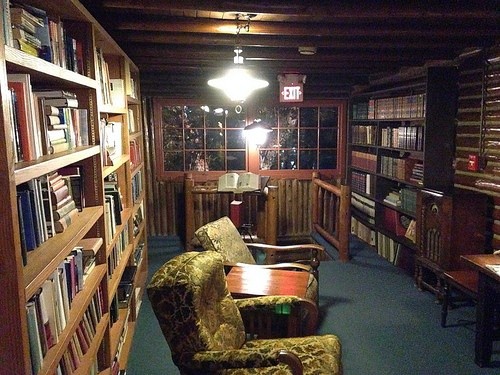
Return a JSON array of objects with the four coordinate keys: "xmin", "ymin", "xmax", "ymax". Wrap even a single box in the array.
[
  {"xmin": 350, "ymin": 92, "xmax": 424, "ymax": 272},
  {"xmin": 217, "ymin": 172, "xmax": 261, "ymax": 193},
  {"xmin": 0, "ymin": 0, "xmax": 146, "ymax": 375},
  {"xmin": 230, "ymin": 200, "xmax": 244, "ymax": 229}
]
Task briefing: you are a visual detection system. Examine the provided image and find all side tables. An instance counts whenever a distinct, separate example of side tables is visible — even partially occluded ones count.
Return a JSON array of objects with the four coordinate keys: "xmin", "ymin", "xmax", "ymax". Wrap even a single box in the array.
[{"xmin": 224, "ymin": 266, "xmax": 310, "ymax": 339}]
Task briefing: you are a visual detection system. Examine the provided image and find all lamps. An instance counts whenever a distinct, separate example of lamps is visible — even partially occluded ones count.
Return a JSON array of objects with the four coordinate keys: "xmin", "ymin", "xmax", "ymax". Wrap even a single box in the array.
[
  {"xmin": 244, "ymin": 89, "xmax": 274, "ymax": 144},
  {"xmin": 208, "ymin": 12, "xmax": 269, "ymax": 100}
]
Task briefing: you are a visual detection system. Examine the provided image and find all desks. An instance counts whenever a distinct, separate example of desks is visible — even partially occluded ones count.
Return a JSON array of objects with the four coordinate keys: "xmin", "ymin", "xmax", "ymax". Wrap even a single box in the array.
[{"xmin": 460, "ymin": 254, "xmax": 500, "ymax": 368}]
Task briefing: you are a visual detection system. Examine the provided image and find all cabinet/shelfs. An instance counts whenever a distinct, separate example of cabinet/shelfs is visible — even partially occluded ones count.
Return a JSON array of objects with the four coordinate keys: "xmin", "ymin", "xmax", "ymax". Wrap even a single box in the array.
[
  {"xmin": 0, "ymin": 0, "xmax": 147, "ymax": 375},
  {"xmin": 346, "ymin": 66, "xmax": 490, "ymax": 301}
]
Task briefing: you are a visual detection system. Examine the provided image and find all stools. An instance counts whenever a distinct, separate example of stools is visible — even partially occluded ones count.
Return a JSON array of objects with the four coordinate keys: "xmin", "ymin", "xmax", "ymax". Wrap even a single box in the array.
[{"xmin": 441, "ymin": 270, "xmax": 479, "ymax": 328}]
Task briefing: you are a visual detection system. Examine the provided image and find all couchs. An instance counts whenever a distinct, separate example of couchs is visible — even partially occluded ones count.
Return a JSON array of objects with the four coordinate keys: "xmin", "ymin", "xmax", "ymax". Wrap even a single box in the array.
[
  {"xmin": 194, "ymin": 216, "xmax": 327, "ymax": 336},
  {"xmin": 147, "ymin": 250, "xmax": 344, "ymax": 375}
]
